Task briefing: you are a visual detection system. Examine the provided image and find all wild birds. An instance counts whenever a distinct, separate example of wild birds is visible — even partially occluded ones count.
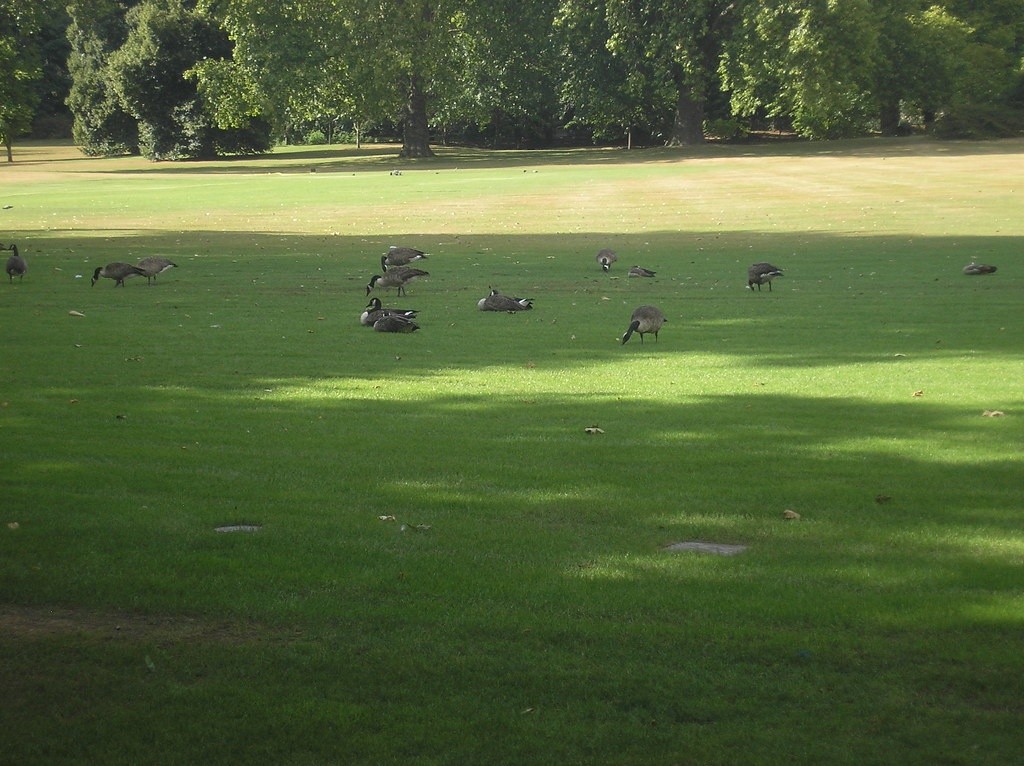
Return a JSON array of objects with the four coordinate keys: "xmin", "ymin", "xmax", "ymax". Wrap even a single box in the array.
[
  {"xmin": 366, "ymin": 266, "xmax": 430, "ymax": 297},
  {"xmin": 381, "ymin": 248, "xmax": 429, "ymax": 273},
  {"xmin": 621, "ymin": 306, "xmax": 670, "ymax": 346},
  {"xmin": 360, "ymin": 298, "xmax": 421, "ymax": 333},
  {"xmin": 389, "ymin": 168, "xmax": 405, "ymax": 176},
  {"xmin": 478, "ymin": 289, "xmax": 536, "ymax": 312},
  {"xmin": 91, "ymin": 258, "xmax": 179, "ymax": 288},
  {"xmin": 0, "ymin": 243, "xmax": 28, "ymax": 282},
  {"xmin": 748, "ymin": 262, "xmax": 784, "ymax": 292},
  {"xmin": 963, "ymin": 262, "xmax": 998, "ymax": 275},
  {"xmin": 628, "ymin": 265, "xmax": 657, "ymax": 278},
  {"xmin": 596, "ymin": 248, "xmax": 617, "ymax": 271}
]
